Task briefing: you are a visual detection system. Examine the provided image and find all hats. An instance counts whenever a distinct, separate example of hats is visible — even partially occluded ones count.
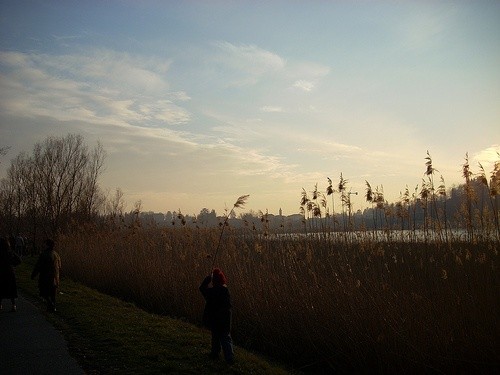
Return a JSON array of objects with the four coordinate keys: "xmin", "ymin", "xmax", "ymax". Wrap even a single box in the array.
[{"xmin": 212, "ymin": 269, "xmax": 226, "ymax": 286}]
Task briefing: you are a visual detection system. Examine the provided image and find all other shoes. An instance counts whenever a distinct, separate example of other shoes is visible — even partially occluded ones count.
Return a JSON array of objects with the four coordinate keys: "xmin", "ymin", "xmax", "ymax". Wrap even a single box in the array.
[
  {"xmin": 53, "ymin": 306, "xmax": 56, "ymax": 312},
  {"xmin": 224, "ymin": 356, "xmax": 236, "ymax": 365},
  {"xmin": 210, "ymin": 352, "xmax": 218, "ymax": 358},
  {"xmin": 32, "ymin": 300, "xmax": 38, "ymax": 303},
  {"xmin": 12, "ymin": 304, "xmax": 17, "ymax": 311},
  {"xmin": 0, "ymin": 305, "xmax": 2, "ymax": 311}
]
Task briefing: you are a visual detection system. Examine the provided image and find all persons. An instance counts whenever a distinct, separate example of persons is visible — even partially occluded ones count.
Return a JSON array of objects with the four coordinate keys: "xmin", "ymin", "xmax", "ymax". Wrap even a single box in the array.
[
  {"xmin": 198, "ymin": 268, "xmax": 236, "ymax": 363},
  {"xmin": 0, "ymin": 237, "xmax": 22, "ymax": 312},
  {"xmin": 30, "ymin": 240, "xmax": 62, "ymax": 324}
]
